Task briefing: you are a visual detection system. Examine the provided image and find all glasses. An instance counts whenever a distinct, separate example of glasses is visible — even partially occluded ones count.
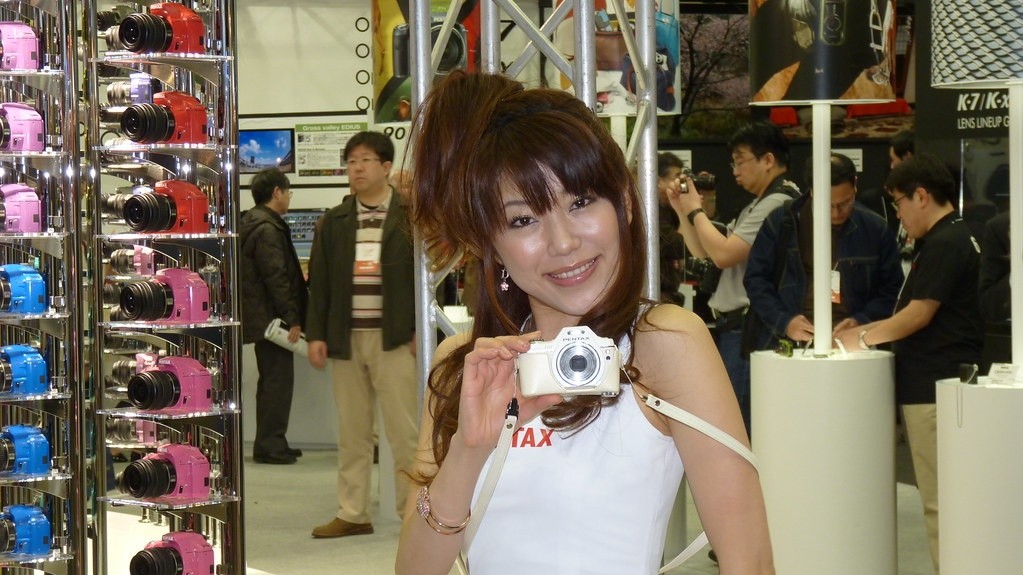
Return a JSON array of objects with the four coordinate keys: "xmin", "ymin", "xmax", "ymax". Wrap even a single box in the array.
[
  {"xmin": 342, "ymin": 157, "xmax": 382, "ymax": 165},
  {"xmin": 283, "ymin": 189, "xmax": 292, "ymax": 197},
  {"xmin": 730, "ymin": 157, "xmax": 756, "ymax": 169},
  {"xmin": 891, "ymin": 193, "xmax": 907, "ymax": 212}
]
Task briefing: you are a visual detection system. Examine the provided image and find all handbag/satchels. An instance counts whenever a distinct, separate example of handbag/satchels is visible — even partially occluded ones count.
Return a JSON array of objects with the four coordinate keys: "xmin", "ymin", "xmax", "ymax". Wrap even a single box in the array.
[
  {"xmin": 654, "ymin": 0, "xmax": 679, "ymax": 63},
  {"xmin": 620, "ymin": 50, "xmax": 676, "ymax": 112}
]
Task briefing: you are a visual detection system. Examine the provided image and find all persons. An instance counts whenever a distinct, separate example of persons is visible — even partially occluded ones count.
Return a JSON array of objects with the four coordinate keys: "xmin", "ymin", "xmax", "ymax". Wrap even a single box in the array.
[
  {"xmin": 889, "ymin": 127, "xmax": 914, "ymax": 170},
  {"xmin": 657, "ymin": 152, "xmax": 708, "ymax": 308},
  {"xmin": 666, "ymin": 121, "xmax": 807, "ymax": 440},
  {"xmin": 307, "ymin": 131, "xmax": 421, "ymax": 541},
  {"xmin": 237, "ymin": 166, "xmax": 310, "ymax": 465},
  {"xmin": 978, "ymin": 210, "xmax": 1012, "ymax": 375},
  {"xmin": 832, "ymin": 153, "xmax": 983, "ymax": 575},
  {"xmin": 394, "ymin": 64, "xmax": 765, "ymax": 575},
  {"xmin": 741, "ymin": 152, "xmax": 906, "ymax": 361}
]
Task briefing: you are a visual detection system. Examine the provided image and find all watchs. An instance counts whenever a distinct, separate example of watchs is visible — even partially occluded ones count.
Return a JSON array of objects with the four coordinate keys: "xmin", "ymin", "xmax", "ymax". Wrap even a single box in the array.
[{"xmin": 858, "ymin": 329, "xmax": 872, "ymax": 350}]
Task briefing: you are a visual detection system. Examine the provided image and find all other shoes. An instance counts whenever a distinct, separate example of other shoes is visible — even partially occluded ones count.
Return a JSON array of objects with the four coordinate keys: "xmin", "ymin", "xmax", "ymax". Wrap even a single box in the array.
[
  {"xmin": 254, "ymin": 448, "xmax": 302, "ymax": 465},
  {"xmin": 312, "ymin": 519, "xmax": 375, "ymax": 538}
]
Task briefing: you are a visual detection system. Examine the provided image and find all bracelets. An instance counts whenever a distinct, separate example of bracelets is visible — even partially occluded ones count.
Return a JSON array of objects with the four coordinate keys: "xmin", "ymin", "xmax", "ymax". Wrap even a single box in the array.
[
  {"xmin": 688, "ymin": 208, "xmax": 708, "ymax": 225},
  {"xmin": 416, "ymin": 482, "xmax": 471, "ymax": 536}
]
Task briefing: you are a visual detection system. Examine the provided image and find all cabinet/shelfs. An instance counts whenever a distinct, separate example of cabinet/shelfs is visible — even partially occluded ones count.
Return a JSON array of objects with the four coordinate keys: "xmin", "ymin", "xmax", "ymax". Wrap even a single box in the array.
[{"xmin": 0, "ymin": 0, "xmax": 245, "ymax": 575}]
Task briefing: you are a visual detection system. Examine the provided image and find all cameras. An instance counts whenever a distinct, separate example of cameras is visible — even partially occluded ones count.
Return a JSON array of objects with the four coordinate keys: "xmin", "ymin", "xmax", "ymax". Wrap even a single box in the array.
[
  {"xmin": 960, "ymin": 364, "xmax": 978, "ymax": 385},
  {"xmin": 0, "ymin": 423, "xmax": 50, "ymax": 474},
  {"xmin": 100, "ymin": 179, "xmax": 209, "ymax": 234},
  {"xmin": 0, "ymin": 182, "xmax": 41, "ymax": 232},
  {"xmin": 680, "ymin": 169, "xmax": 716, "ymax": 193},
  {"xmin": 107, "ymin": 72, "xmax": 207, "ymax": 144},
  {"xmin": 0, "ymin": 344, "xmax": 48, "ymax": 395},
  {"xmin": 101, "ymin": 243, "xmax": 210, "ymax": 323},
  {"xmin": 104, "ymin": 418, "xmax": 209, "ymax": 499},
  {"xmin": 129, "ymin": 531, "xmax": 214, "ymax": 575},
  {"xmin": 0, "ymin": 102, "xmax": 44, "ymax": 151},
  {"xmin": 773, "ymin": 339, "xmax": 793, "ymax": 357},
  {"xmin": 0, "ymin": 20, "xmax": 39, "ymax": 69},
  {"xmin": 819, "ymin": 0, "xmax": 846, "ymax": 46},
  {"xmin": 0, "ymin": 263, "xmax": 47, "ymax": 313},
  {"xmin": 514, "ymin": 326, "xmax": 622, "ymax": 398},
  {"xmin": 0, "ymin": 503, "xmax": 51, "ymax": 555},
  {"xmin": 97, "ymin": 2, "xmax": 205, "ymax": 54},
  {"xmin": 392, "ymin": 12, "xmax": 468, "ymax": 78},
  {"xmin": 105, "ymin": 328, "xmax": 212, "ymax": 411}
]
